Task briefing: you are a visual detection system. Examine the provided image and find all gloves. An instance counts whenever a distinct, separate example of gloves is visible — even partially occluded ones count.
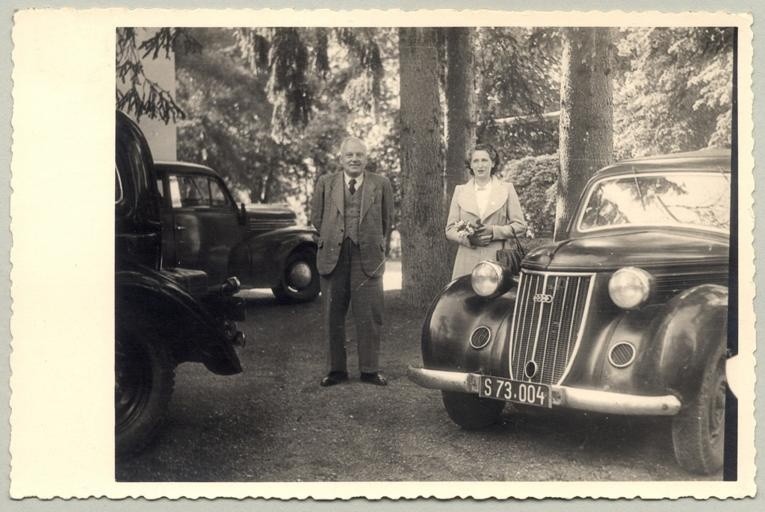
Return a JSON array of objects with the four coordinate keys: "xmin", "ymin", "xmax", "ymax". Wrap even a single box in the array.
[{"xmin": 469, "ymin": 219, "xmax": 494, "ymax": 246}]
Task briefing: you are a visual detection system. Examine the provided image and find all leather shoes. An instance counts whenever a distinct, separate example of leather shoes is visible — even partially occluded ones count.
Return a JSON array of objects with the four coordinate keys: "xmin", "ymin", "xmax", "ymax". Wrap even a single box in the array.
[
  {"xmin": 361, "ymin": 373, "xmax": 386, "ymax": 385},
  {"xmin": 321, "ymin": 373, "xmax": 347, "ymax": 385}
]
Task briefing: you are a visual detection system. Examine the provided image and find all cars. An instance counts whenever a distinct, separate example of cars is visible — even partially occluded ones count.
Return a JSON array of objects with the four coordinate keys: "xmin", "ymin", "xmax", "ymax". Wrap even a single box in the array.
[
  {"xmin": 398, "ymin": 145, "xmax": 733, "ymax": 477},
  {"xmin": 154, "ymin": 159, "xmax": 321, "ymax": 306},
  {"xmin": 115, "ymin": 105, "xmax": 248, "ymax": 460}
]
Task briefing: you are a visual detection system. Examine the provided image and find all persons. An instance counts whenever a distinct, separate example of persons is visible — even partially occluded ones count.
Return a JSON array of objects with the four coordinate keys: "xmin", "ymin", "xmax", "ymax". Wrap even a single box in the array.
[
  {"xmin": 443, "ymin": 143, "xmax": 530, "ymax": 283},
  {"xmin": 309, "ymin": 136, "xmax": 395, "ymax": 386}
]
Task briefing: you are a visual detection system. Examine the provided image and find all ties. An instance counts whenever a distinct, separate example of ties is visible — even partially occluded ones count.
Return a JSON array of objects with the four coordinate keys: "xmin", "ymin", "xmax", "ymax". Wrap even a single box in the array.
[{"xmin": 349, "ymin": 179, "xmax": 356, "ymax": 193}]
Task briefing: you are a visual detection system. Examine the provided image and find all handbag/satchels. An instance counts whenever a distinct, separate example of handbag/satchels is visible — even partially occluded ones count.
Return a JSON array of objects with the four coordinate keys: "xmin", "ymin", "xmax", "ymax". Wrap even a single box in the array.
[{"xmin": 496, "ymin": 248, "xmax": 530, "ymax": 274}]
